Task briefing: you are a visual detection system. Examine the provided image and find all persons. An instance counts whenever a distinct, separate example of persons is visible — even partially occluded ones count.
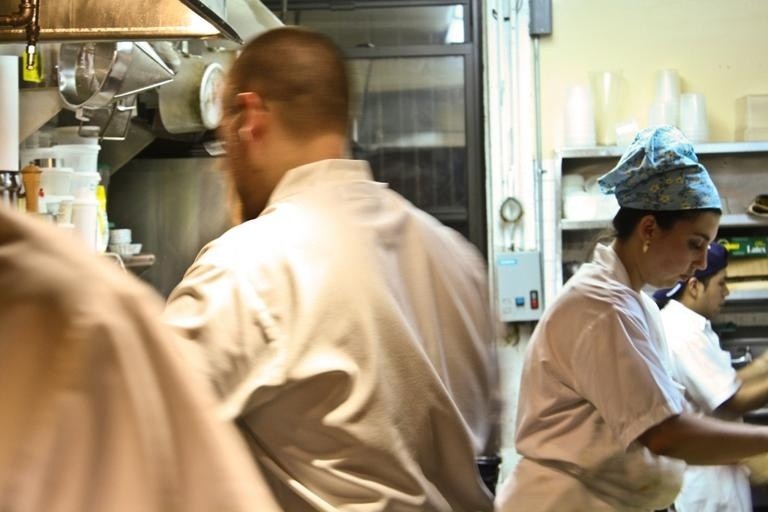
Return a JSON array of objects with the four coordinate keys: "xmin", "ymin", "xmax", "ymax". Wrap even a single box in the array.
[
  {"xmin": 651, "ymin": 235, "xmax": 767, "ymax": 512},
  {"xmin": 496, "ymin": 120, "xmax": 768, "ymax": 512},
  {"xmin": 166, "ymin": 26, "xmax": 504, "ymax": 512},
  {"xmin": 1, "ymin": 195, "xmax": 287, "ymax": 510}
]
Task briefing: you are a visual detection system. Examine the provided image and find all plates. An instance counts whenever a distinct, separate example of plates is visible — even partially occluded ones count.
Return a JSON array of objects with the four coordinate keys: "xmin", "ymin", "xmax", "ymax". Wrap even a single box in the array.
[{"xmin": 110, "ymin": 243, "xmax": 142, "ymax": 256}]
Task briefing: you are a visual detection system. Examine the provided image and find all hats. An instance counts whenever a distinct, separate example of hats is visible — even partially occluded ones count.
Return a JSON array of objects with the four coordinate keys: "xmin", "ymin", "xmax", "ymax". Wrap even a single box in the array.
[
  {"xmin": 654, "ymin": 241, "xmax": 727, "ymax": 302},
  {"xmin": 597, "ymin": 126, "xmax": 722, "ymax": 211}
]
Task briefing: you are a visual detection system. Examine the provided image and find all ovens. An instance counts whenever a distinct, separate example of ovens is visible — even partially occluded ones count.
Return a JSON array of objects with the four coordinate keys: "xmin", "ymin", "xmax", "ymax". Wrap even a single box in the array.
[{"xmin": 706, "ymin": 287, "xmax": 768, "ymax": 420}]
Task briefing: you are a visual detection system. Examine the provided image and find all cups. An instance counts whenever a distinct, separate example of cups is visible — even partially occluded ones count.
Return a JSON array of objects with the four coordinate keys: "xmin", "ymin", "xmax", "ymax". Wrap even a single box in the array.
[
  {"xmin": 559, "ymin": 173, "xmax": 620, "ymax": 223},
  {"xmin": 24, "ymin": 126, "xmax": 109, "ymax": 260},
  {"xmin": 646, "ymin": 69, "xmax": 710, "ymax": 144},
  {"xmin": 110, "ymin": 229, "xmax": 132, "ymax": 245}
]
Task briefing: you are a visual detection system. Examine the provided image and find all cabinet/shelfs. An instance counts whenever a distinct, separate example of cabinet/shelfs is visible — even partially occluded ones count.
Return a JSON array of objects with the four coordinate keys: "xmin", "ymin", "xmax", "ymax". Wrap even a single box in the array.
[{"xmin": 555, "ymin": 151, "xmax": 768, "ymax": 420}]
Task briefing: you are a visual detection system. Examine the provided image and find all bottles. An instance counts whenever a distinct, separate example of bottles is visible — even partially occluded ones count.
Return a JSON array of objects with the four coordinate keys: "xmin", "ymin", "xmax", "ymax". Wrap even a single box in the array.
[{"xmin": 593, "ymin": 65, "xmax": 620, "ymax": 146}]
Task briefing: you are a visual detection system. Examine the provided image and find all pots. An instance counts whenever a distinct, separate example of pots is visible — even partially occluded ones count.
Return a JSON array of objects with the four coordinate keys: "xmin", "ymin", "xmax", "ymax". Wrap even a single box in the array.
[{"xmin": 158, "ymin": 38, "xmax": 227, "ymax": 135}]
